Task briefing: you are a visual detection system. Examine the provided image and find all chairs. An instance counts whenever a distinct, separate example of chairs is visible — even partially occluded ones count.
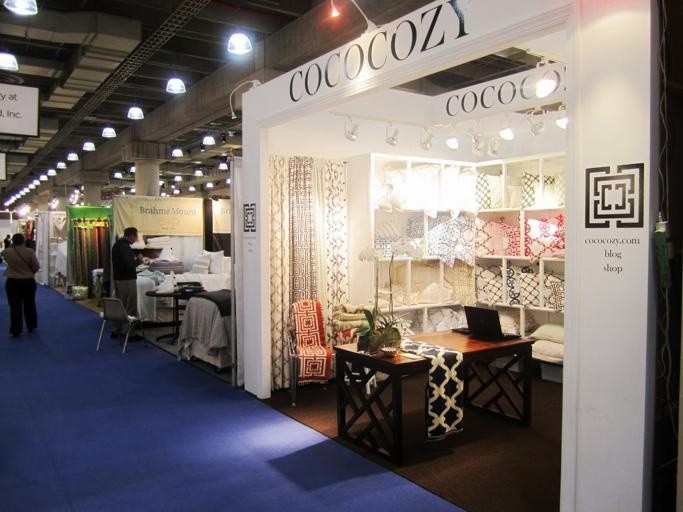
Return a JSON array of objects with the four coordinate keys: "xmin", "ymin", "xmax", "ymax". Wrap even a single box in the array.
[
  {"xmin": 96, "ymin": 298, "xmax": 149, "ymax": 354},
  {"xmin": 287, "ymin": 299, "xmax": 336, "ymax": 406}
]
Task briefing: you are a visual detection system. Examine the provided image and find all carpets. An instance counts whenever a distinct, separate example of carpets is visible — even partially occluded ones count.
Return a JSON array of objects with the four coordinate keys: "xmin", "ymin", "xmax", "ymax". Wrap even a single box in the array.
[{"xmin": 50, "ymin": 285, "xmax": 562, "ymax": 512}]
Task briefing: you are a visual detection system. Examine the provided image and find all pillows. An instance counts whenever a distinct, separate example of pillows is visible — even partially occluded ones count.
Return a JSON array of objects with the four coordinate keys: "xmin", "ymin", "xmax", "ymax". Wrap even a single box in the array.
[
  {"xmin": 191, "ymin": 248, "xmax": 231, "ymax": 276},
  {"xmin": 375, "ymin": 164, "xmax": 563, "ymax": 361}
]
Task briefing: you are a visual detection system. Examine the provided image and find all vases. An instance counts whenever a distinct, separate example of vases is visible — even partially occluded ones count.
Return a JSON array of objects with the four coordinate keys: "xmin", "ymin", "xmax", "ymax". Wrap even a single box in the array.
[{"xmin": 356, "ymin": 328, "xmax": 405, "ymax": 355}]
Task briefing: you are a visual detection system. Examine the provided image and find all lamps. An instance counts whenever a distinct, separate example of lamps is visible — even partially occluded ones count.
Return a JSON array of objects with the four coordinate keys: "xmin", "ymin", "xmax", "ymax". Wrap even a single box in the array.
[
  {"xmin": 4, "ymin": 162, "xmax": 67, "ymax": 208},
  {"xmin": 81, "ymin": 130, "xmax": 96, "ymax": 152},
  {"xmin": 102, "ymin": 112, "xmax": 117, "ymax": 140},
  {"xmin": 171, "ymin": 141, "xmax": 184, "ymax": 158},
  {"xmin": 327, "ymin": 2, "xmax": 344, "ymax": 21},
  {"xmin": 68, "ymin": 186, "xmax": 86, "ymax": 207},
  {"xmin": 160, "ymin": 54, "xmax": 189, "ymax": 96},
  {"xmin": 113, "ymin": 169, "xmax": 123, "ymax": 179},
  {"xmin": 126, "ymin": 91, "xmax": 146, "ymax": 125},
  {"xmin": 222, "ymin": 3, "xmax": 257, "ymax": 59},
  {"xmin": 66, "ymin": 139, "xmax": 80, "ymax": 161},
  {"xmin": 122, "ymin": 189, "xmax": 137, "ymax": 195},
  {"xmin": 202, "ymin": 127, "xmax": 217, "ymax": 147},
  {"xmin": 158, "ymin": 163, "xmax": 232, "ymax": 197},
  {"xmin": 130, "ymin": 161, "xmax": 137, "ymax": 174},
  {"xmin": 329, "ymin": 47, "xmax": 568, "ymax": 155}
]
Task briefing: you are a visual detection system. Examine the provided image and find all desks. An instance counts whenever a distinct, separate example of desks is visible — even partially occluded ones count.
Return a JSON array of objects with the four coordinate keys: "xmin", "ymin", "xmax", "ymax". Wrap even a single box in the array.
[
  {"xmin": 176, "ymin": 288, "xmax": 231, "ymax": 372},
  {"xmin": 131, "ymin": 247, "xmax": 163, "ymax": 258},
  {"xmin": 146, "ymin": 288, "xmax": 209, "ymax": 345},
  {"xmin": 333, "ymin": 331, "xmax": 537, "ymax": 467}
]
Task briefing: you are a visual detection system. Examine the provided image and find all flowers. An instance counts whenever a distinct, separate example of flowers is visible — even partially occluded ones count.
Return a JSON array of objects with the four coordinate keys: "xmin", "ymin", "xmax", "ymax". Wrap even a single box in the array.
[{"xmin": 355, "ymin": 234, "xmax": 427, "ymax": 354}]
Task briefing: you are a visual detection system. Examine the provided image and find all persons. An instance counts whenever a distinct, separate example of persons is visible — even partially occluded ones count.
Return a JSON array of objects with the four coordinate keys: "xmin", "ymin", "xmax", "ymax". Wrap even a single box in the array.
[
  {"xmin": 25, "ymin": 236, "xmax": 35, "ymax": 249},
  {"xmin": 2, "ymin": 234, "xmax": 40, "ymax": 337},
  {"xmin": 111, "ymin": 227, "xmax": 145, "ymax": 338},
  {"xmin": 4, "ymin": 235, "xmax": 12, "ymax": 248}
]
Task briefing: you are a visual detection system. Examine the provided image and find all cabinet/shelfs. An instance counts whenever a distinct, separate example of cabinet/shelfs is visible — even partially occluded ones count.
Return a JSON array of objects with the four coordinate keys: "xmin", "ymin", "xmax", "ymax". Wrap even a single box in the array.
[{"xmin": 346, "ymin": 149, "xmax": 564, "ymax": 384}]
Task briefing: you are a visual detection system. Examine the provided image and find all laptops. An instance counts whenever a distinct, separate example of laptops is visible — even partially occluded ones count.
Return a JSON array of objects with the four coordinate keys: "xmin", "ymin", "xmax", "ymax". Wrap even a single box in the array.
[{"xmin": 465, "ymin": 306, "xmax": 522, "ymax": 343}]
